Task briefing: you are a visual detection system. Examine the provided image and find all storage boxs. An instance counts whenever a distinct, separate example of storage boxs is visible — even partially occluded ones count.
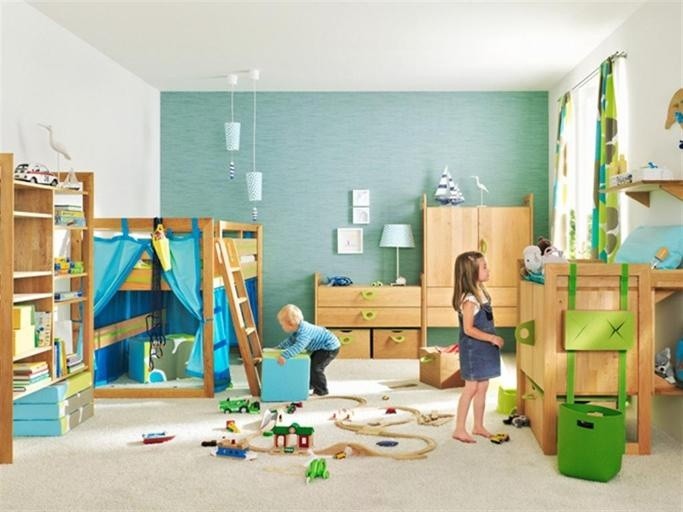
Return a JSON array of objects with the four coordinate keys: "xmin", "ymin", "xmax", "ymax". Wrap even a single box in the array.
[
  {"xmin": 329, "ymin": 329, "xmax": 371, "ymax": 359},
  {"xmin": 419, "ymin": 346, "xmax": 466, "ymax": 390},
  {"xmin": 373, "ymin": 329, "xmax": 421, "ymax": 359}
]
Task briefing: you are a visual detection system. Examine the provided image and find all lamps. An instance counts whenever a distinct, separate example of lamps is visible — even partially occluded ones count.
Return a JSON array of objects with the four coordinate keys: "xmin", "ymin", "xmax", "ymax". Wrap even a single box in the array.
[
  {"xmin": 245, "ymin": 69, "xmax": 264, "ymax": 223},
  {"xmin": 378, "ymin": 223, "xmax": 416, "ymax": 287},
  {"xmin": 224, "ymin": 75, "xmax": 241, "ymax": 181}
]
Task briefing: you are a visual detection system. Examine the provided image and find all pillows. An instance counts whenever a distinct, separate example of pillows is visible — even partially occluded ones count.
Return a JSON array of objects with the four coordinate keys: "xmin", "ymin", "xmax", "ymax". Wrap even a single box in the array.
[{"xmin": 613, "ymin": 224, "xmax": 683, "ymax": 270}]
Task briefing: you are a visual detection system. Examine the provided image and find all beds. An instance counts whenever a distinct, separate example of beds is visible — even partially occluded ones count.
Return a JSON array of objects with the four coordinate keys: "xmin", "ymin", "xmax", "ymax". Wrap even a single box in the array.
[
  {"xmin": 69, "ymin": 216, "xmax": 265, "ymax": 399},
  {"xmin": 514, "ymin": 257, "xmax": 683, "ymax": 457}
]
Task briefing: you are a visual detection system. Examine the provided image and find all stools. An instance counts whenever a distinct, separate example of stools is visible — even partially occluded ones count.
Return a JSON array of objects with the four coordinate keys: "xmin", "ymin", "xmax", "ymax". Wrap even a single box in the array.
[
  {"xmin": 260, "ymin": 347, "xmax": 312, "ymax": 403},
  {"xmin": 165, "ymin": 333, "xmax": 197, "ymax": 379},
  {"xmin": 128, "ymin": 337, "xmax": 176, "ymax": 384}
]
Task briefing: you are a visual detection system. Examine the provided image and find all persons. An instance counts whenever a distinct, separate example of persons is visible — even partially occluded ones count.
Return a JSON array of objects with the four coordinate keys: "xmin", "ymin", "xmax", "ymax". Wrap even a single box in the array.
[
  {"xmin": 274, "ymin": 304, "xmax": 341, "ymax": 397},
  {"xmin": 452, "ymin": 252, "xmax": 504, "ymax": 443}
]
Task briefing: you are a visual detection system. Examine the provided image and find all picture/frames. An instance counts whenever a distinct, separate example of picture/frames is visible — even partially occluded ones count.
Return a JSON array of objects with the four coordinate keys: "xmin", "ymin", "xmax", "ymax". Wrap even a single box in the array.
[
  {"xmin": 337, "ymin": 228, "xmax": 363, "ymax": 254},
  {"xmin": 352, "ymin": 190, "xmax": 370, "ymax": 207},
  {"xmin": 352, "ymin": 207, "xmax": 370, "ymax": 225}
]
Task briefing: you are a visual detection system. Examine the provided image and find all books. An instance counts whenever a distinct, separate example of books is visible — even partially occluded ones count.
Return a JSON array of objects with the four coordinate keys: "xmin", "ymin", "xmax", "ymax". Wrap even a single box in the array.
[{"xmin": 13, "ymin": 205, "xmax": 86, "ymax": 392}]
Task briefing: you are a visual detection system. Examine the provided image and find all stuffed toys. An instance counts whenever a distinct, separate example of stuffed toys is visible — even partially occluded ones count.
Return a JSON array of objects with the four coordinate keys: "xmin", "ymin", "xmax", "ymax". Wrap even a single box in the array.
[
  {"xmin": 518, "ymin": 236, "xmax": 552, "ymax": 276},
  {"xmin": 523, "ymin": 245, "xmax": 566, "ymax": 274}
]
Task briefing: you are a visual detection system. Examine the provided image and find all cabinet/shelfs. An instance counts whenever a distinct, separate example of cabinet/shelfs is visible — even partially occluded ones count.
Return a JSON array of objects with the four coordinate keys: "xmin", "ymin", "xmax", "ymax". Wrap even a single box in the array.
[
  {"xmin": 314, "ymin": 272, "xmax": 424, "ymax": 346},
  {"xmin": 424, "ymin": 193, "xmax": 534, "ymax": 346},
  {"xmin": 0, "ymin": 152, "xmax": 96, "ymax": 464}
]
